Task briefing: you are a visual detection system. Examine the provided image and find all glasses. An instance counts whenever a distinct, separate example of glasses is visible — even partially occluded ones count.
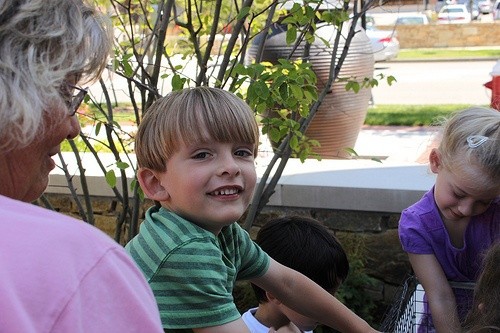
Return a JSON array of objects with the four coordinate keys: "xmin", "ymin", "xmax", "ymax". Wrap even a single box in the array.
[{"xmin": 60, "ymin": 81, "xmax": 89, "ymax": 116}]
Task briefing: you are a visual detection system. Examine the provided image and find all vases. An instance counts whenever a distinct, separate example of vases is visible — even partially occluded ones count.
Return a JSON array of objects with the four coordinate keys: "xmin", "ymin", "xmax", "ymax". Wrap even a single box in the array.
[{"xmin": 247, "ymin": 7, "xmax": 374, "ymax": 159}]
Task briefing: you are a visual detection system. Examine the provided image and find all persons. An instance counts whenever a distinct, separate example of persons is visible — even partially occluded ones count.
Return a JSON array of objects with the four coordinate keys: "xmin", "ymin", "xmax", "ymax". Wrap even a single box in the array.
[
  {"xmin": 398, "ymin": 106, "xmax": 500, "ymax": 332},
  {"xmin": 1, "ymin": 0, "xmax": 165, "ymax": 333},
  {"xmin": 124, "ymin": 87, "xmax": 382, "ymax": 332},
  {"xmin": 242, "ymin": 216, "xmax": 348, "ymax": 333}
]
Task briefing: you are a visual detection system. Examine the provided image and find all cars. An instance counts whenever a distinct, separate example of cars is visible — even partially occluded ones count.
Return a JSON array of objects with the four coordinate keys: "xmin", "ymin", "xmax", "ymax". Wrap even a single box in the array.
[
  {"xmin": 372, "ymin": 30, "xmax": 399, "ymax": 62},
  {"xmin": 395, "ymin": 17, "xmax": 427, "ymax": 27},
  {"xmin": 438, "ymin": 6, "xmax": 471, "ymax": 24},
  {"xmin": 468, "ymin": 1, "xmax": 499, "ymax": 20}
]
